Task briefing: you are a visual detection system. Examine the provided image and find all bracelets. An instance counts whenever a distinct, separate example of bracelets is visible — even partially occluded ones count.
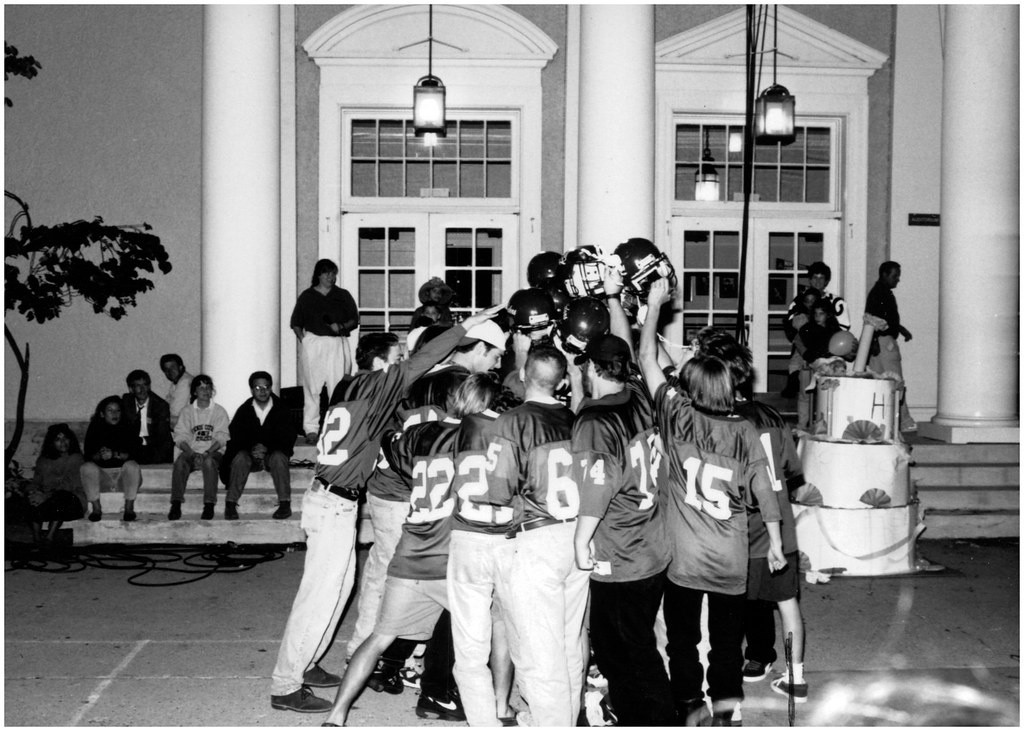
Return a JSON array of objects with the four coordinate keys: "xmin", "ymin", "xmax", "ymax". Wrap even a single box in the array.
[{"xmin": 606, "ymin": 294, "xmax": 620, "ymax": 299}]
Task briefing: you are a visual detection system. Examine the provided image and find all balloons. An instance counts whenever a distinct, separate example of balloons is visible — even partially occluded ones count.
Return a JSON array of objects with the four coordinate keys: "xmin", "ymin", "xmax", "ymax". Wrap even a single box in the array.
[{"xmin": 829, "ymin": 331, "xmax": 854, "ymax": 356}]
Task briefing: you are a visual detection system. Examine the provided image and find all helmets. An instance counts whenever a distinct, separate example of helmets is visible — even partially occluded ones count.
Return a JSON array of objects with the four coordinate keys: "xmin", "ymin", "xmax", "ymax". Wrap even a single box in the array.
[{"xmin": 502, "ymin": 239, "xmax": 677, "ymax": 351}]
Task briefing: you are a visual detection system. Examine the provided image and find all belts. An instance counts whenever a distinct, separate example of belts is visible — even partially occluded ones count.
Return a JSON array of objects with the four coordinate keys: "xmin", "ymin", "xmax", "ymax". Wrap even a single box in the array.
[
  {"xmin": 315, "ymin": 476, "xmax": 361, "ymax": 502},
  {"xmin": 516, "ymin": 517, "xmax": 575, "ymax": 532}
]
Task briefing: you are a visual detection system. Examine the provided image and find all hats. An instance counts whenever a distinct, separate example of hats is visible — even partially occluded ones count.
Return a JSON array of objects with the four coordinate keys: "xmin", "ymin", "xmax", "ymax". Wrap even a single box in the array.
[
  {"xmin": 408, "ymin": 325, "xmax": 428, "ymax": 350},
  {"xmin": 455, "ymin": 318, "xmax": 507, "ymax": 352},
  {"xmin": 574, "ymin": 334, "xmax": 631, "ymax": 366},
  {"xmin": 807, "ymin": 262, "xmax": 831, "ymax": 286}
]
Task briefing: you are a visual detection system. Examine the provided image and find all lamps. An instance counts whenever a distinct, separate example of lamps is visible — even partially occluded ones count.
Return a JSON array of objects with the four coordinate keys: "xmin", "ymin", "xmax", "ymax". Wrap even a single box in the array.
[
  {"xmin": 412, "ymin": 4, "xmax": 447, "ymax": 140},
  {"xmin": 695, "ymin": 124, "xmax": 720, "ymax": 205},
  {"xmin": 755, "ymin": 6, "xmax": 797, "ymax": 149}
]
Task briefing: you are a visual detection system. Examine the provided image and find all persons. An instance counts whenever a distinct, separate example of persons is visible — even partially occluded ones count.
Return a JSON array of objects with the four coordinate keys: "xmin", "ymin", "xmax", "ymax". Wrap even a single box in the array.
[
  {"xmin": 782, "ymin": 269, "xmax": 859, "ymax": 432},
  {"xmin": 28, "ymin": 423, "xmax": 88, "ymax": 552},
  {"xmin": 290, "ymin": 258, "xmax": 359, "ymax": 444},
  {"xmin": 270, "ymin": 268, "xmax": 809, "ymax": 727},
  {"xmin": 168, "ymin": 375, "xmax": 230, "ymax": 520},
  {"xmin": 80, "ymin": 395, "xmax": 142, "ymax": 521},
  {"xmin": 219, "ymin": 372, "xmax": 293, "ymax": 520},
  {"xmin": 866, "ymin": 260, "xmax": 918, "ymax": 432},
  {"xmin": 121, "ymin": 369, "xmax": 174, "ymax": 464},
  {"xmin": 160, "ymin": 354, "xmax": 194, "ymax": 431}
]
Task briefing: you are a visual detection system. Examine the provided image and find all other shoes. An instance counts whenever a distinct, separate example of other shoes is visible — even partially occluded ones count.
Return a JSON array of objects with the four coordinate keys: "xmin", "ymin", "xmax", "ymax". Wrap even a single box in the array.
[
  {"xmin": 123, "ymin": 509, "xmax": 136, "ymax": 521},
  {"xmin": 273, "ymin": 506, "xmax": 292, "ymax": 519},
  {"xmin": 499, "ymin": 704, "xmax": 520, "ymax": 726},
  {"xmin": 515, "ymin": 711, "xmax": 534, "ymax": 727},
  {"xmin": 88, "ymin": 511, "xmax": 103, "ymax": 522},
  {"xmin": 201, "ymin": 508, "xmax": 214, "ymax": 519},
  {"xmin": 225, "ymin": 508, "xmax": 240, "ymax": 519},
  {"xmin": 306, "ymin": 433, "xmax": 318, "ymax": 445},
  {"xmin": 168, "ymin": 508, "xmax": 181, "ymax": 520}
]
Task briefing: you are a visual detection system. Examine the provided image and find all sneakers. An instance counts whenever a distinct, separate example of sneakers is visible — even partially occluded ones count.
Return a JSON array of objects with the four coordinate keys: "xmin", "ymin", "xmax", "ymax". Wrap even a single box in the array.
[
  {"xmin": 344, "ymin": 655, "xmax": 422, "ymax": 694},
  {"xmin": 416, "ymin": 691, "xmax": 466, "ymax": 723},
  {"xmin": 271, "ymin": 685, "xmax": 332, "ymax": 713},
  {"xmin": 770, "ymin": 677, "xmax": 808, "ymax": 702},
  {"xmin": 574, "ymin": 665, "xmax": 743, "ymax": 726},
  {"xmin": 742, "ymin": 660, "xmax": 773, "ymax": 682},
  {"xmin": 303, "ymin": 663, "xmax": 341, "ymax": 688}
]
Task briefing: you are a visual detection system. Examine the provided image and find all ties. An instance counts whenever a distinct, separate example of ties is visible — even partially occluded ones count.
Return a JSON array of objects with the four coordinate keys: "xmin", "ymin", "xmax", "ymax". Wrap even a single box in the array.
[{"xmin": 133, "ymin": 404, "xmax": 145, "ymax": 439}]
